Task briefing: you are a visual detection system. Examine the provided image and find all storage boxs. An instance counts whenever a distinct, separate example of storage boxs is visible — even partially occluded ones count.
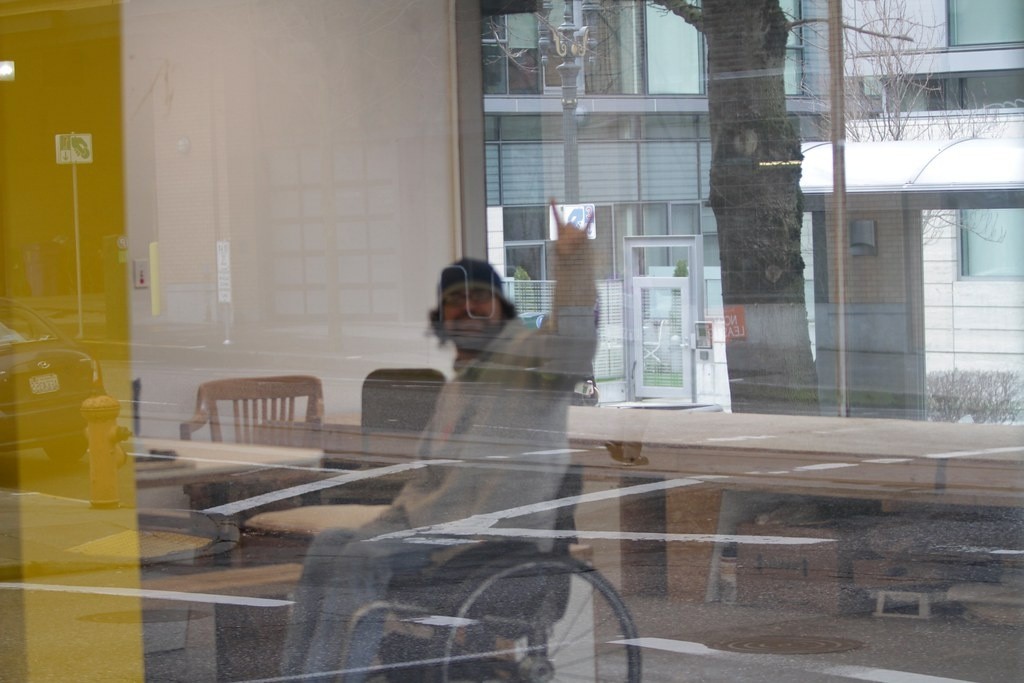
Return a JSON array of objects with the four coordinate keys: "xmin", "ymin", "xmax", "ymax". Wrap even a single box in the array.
[{"xmin": 736, "ymin": 517, "xmax": 874, "ymax": 618}]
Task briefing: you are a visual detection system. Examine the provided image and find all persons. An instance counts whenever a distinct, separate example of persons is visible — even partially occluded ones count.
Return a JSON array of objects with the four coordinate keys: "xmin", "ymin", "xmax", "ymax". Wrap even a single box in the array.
[{"xmin": 280, "ymin": 195, "xmax": 600, "ymax": 683}]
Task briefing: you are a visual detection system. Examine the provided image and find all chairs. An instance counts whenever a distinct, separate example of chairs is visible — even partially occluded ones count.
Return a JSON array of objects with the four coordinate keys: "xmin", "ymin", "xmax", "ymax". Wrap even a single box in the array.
[{"xmin": 181, "ymin": 362, "xmax": 455, "ymax": 566}]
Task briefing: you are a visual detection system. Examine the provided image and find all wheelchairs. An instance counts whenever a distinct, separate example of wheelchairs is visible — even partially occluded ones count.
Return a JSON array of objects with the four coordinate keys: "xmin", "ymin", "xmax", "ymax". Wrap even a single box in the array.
[{"xmin": 332, "ymin": 448, "xmax": 642, "ymax": 683}]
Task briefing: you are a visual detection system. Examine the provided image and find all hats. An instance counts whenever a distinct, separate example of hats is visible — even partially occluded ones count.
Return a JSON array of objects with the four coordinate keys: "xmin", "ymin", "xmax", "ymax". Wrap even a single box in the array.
[{"xmin": 439, "ymin": 257, "xmax": 505, "ymax": 298}]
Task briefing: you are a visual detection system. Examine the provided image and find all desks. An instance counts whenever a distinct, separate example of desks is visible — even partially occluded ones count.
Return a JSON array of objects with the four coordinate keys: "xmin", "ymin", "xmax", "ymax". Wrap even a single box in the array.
[{"xmin": 130, "ymin": 430, "xmax": 324, "ymax": 491}]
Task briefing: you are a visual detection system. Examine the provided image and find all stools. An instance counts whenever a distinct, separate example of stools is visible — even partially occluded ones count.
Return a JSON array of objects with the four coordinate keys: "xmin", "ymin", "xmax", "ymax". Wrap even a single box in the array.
[{"xmin": 144, "ymin": 560, "xmax": 305, "ymax": 683}]
